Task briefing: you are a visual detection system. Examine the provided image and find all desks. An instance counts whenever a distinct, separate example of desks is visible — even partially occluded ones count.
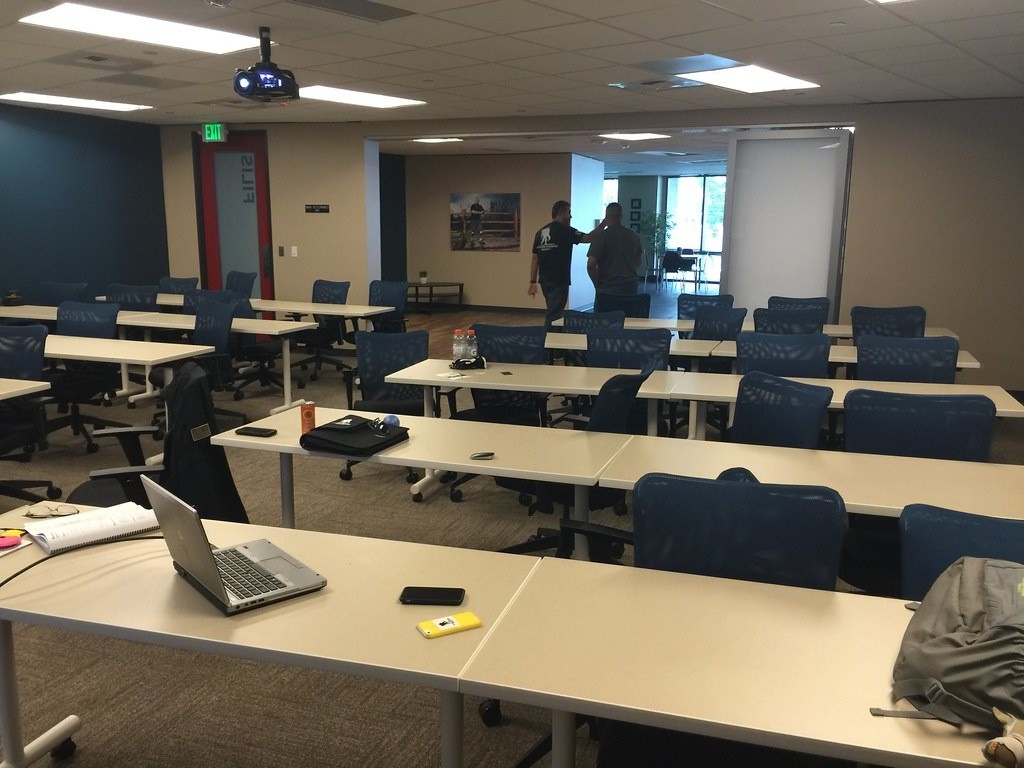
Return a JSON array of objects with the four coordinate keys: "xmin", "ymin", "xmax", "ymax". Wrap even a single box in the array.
[
  {"xmin": 460, "ymin": 555, "xmax": 1010, "ymax": 768},
  {"xmin": 117, "ymin": 312, "xmax": 319, "ymax": 416},
  {"xmin": 44, "ymin": 334, "xmax": 216, "ymax": 466},
  {"xmin": 243, "ymin": 298, "xmax": 396, "ymax": 390},
  {"xmin": 658, "ymin": 255, "xmax": 702, "ymax": 294},
  {"xmin": 1, "ymin": 378, "xmax": 52, "ymax": 401},
  {"xmin": 0, "ymin": 304, "xmax": 160, "ymax": 407},
  {"xmin": 599, "ymin": 432, "xmax": 1024, "ymax": 522},
  {"xmin": 670, "ymin": 369, "xmax": 1024, "ymax": 442},
  {"xmin": 210, "ymin": 403, "xmax": 634, "ymax": 566},
  {"xmin": 551, "ymin": 317, "xmax": 960, "ymax": 345},
  {"xmin": 94, "ymin": 288, "xmax": 262, "ymax": 307},
  {"xmin": 385, "ymin": 359, "xmax": 685, "ymax": 503},
  {"xmin": 0, "ymin": 499, "xmax": 543, "ymax": 768},
  {"xmin": 407, "ymin": 282, "xmax": 465, "ymax": 316}
]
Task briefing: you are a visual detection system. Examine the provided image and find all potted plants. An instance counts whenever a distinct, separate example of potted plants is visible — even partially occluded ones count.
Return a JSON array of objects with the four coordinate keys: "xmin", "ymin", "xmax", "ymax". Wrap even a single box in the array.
[
  {"xmin": 419, "ymin": 271, "xmax": 428, "ymax": 285},
  {"xmin": 640, "ymin": 210, "xmax": 677, "ymax": 283}
]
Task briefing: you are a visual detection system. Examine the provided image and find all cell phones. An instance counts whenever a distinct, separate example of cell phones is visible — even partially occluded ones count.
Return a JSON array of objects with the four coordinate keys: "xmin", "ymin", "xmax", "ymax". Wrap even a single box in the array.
[
  {"xmin": 416, "ymin": 611, "xmax": 481, "ymax": 638},
  {"xmin": 399, "ymin": 586, "xmax": 465, "ymax": 606},
  {"xmin": 235, "ymin": 427, "xmax": 277, "ymax": 437}
]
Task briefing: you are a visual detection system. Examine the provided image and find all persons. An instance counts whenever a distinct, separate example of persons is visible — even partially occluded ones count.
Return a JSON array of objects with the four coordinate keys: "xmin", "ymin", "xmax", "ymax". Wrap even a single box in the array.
[
  {"xmin": 528, "ymin": 201, "xmax": 606, "ymax": 319},
  {"xmin": 587, "ymin": 203, "xmax": 642, "ymax": 312},
  {"xmin": 471, "ymin": 197, "xmax": 487, "ymax": 244}
]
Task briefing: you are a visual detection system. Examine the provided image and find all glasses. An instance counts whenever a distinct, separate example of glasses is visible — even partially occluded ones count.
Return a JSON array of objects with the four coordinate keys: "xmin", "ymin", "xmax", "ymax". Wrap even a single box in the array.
[{"xmin": 23, "ymin": 504, "xmax": 79, "ymax": 518}]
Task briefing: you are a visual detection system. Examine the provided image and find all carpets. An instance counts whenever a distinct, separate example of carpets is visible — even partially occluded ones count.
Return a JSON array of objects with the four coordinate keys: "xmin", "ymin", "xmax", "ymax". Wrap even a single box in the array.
[{"xmin": 0, "ymin": 310, "xmax": 868, "ymax": 767}]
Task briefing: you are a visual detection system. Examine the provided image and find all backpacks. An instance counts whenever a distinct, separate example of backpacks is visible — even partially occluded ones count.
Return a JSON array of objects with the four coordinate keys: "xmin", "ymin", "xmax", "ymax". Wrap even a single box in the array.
[{"xmin": 869, "ymin": 556, "xmax": 1024, "ymax": 730}]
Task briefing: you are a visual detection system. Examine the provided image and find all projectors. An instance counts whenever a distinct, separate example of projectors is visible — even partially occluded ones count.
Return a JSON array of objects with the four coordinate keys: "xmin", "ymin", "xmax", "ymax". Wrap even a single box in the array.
[{"xmin": 233, "ymin": 67, "xmax": 300, "ymax": 103}]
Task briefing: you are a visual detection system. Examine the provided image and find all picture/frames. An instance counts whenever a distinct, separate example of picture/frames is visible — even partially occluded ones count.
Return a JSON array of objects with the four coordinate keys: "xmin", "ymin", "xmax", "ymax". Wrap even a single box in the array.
[
  {"xmin": 630, "ymin": 223, "xmax": 640, "ymax": 233},
  {"xmin": 631, "ymin": 211, "xmax": 641, "ymax": 221},
  {"xmin": 631, "ymin": 199, "xmax": 641, "ymax": 209}
]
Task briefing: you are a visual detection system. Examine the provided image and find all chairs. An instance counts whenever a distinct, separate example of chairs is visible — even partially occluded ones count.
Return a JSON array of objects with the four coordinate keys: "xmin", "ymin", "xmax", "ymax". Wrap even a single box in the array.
[
  {"xmin": 661, "ymin": 308, "xmax": 747, "ymax": 434},
  {"xmin": 900, "ymin": 504, "xmax": 1024, "ymax": 601},
  {"xmin": 338, "ymin": 328, "xmax": 431, "ymax": 483},
  {"xmin": 0, "ymin": 270, "xmax": 414, "ymax": 504},
  {"xmin": 857, "ymin": 333, "xmax": 961, "ymax": 383},
  {"xmin": 563, "ymin": 309, "xmax": 626, "ymax": 366},
  {"xmin": 493, "ymin": 356, "xmax": 659, "ymax": 567},
  {"xmin": 642, "ymin": 247, "xmax": 710, "ymax": 293},
  {"xmin": 668, "ymin": 293, "xmax": 734, "ymax": 372},
  {"xmin": 752, "ymin": 307, "xmax": 826, "ymax": 336},
  {"xmin": 595, "ymin": 471, "xmax": 851, "ymax": 767},
  {"xmin": 836, "ymin": 387, "xmax": 998, "ymax": 599},
  {"xmin": 64, "ymin": 361, "xmax": 251, "ymax": 525},
  {"xmin": 768, "ymin": 295, "xmax": 832, "ymax": 324},
  {"xmin": 720, "ymin": 370, "xmax": 834, "ymax": 449},
  {"xmin": 437, "ymin": 324, "xmax": 554, "ymax": 503},
  {"xmin": 549, "ymin": 327, "xmax": 673, "ymax": 436},
  {"xmin": 850, "ymin": 305, "xmax": 926, "ymax": 347},
  {"xmin": 735, "ymin": 331, "xmax": 833, "ymax": 379},
  {"xmin": 593, "ymin": 293, "xmax": 652, "ymax": 319}
]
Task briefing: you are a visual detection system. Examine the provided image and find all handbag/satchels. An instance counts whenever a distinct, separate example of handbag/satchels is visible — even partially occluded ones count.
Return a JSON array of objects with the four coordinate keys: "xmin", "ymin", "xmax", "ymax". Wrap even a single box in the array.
[{"xmin": 300, "ymin": 415, "xmax": 410, "ymax": 457}]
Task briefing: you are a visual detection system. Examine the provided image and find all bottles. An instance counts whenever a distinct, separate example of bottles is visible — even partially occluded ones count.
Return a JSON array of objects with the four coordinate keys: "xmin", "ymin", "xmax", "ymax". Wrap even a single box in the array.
[
  {"xmin": 453, "ymin": 328, "xmax": 467, "ymax": 359},
  {"xmin": 466, "ymin": 330, "xmax": 478, "ymax": 359}
]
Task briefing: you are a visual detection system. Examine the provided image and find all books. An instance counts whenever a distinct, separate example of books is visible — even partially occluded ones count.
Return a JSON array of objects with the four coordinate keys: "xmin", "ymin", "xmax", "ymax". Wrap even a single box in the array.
[{"xmin": 22, "ymin": 501, "xmax": 160, "ymax": 556}]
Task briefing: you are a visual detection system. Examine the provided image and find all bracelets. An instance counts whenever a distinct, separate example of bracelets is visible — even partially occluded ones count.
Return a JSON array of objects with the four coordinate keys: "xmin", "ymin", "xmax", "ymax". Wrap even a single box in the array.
[{"xmin": 530, "ymin": 280, "xmax": 536, "ymax": 283}]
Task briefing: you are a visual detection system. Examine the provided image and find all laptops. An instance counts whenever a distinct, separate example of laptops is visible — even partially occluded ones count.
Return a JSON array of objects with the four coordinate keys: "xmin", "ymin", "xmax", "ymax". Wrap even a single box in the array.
[{"xmin": 140, "ymin": 474, "xmax": 328, "ymax": 616}]
